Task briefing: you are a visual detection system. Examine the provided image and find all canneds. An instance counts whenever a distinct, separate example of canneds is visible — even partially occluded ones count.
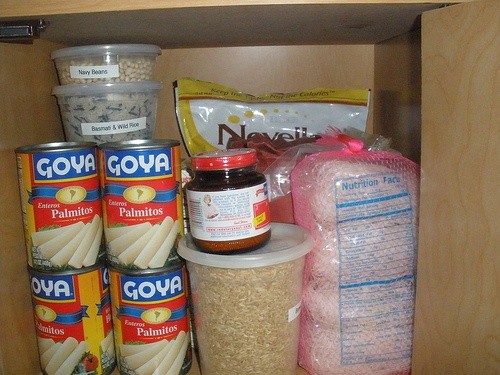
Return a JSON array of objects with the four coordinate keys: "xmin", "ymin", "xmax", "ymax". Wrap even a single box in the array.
[
  {"xmin": 183, "ymin": 149, "xmax": 272, "ymax": 255},
  {"xmin": 106, "ymin": 258, "xmax": 192, "ymax": 375},
  {"xmin": 14, "ymin": 141, "xmax": 106, "ymax": 275},
  {"xmin": 26, "ymin": 262, "xmax": 117, "ymax": 375},
  {"xmin": 96, "ymin": 139, "xmax": 185, "ymax": 276}
]
